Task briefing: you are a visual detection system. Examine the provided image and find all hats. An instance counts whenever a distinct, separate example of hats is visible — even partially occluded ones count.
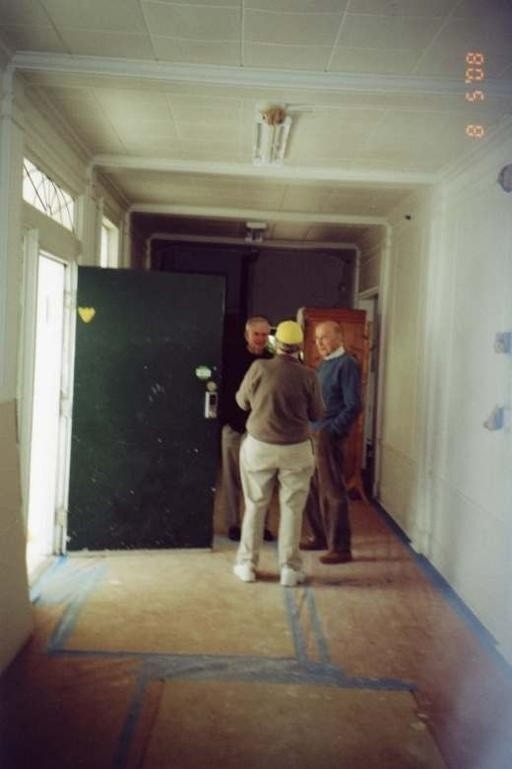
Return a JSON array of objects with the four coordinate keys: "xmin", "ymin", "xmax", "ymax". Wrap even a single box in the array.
[{"xmin": 275, "ymin": 320, "xmax": 304, "ymax": 343}]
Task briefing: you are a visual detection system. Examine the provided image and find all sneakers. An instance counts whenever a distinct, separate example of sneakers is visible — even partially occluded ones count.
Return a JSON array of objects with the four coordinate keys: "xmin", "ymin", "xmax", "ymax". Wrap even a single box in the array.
[{"xmin": 229, "ymin": 525, "xmax": 352, "ymax": 585}]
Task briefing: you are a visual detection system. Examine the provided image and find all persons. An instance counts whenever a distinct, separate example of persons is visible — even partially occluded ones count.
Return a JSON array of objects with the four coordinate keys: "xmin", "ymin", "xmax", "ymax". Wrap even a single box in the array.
[
  {"xmin": 219, "ymin": 316, "xmax": 275, "ymax": 542},
  {"xmin": 234, "ymin": 320, "xmax": 324, "ymax": 587},
  {"xmin": 299, "ymin": 320, "xmax": 364, "ymax": 563}
]
